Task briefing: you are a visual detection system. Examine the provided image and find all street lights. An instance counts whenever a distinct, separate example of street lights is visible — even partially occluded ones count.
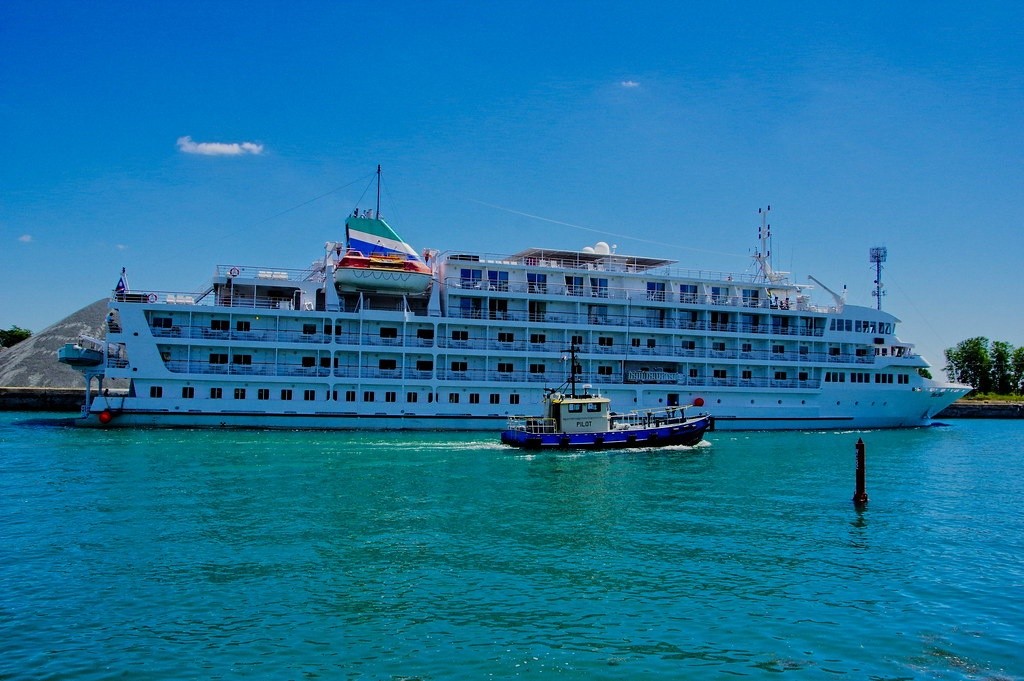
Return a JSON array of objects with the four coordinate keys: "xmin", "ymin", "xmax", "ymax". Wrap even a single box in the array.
[{"xmin": 948, "ymin": 361, "xmax": 958, "ymax": 383}]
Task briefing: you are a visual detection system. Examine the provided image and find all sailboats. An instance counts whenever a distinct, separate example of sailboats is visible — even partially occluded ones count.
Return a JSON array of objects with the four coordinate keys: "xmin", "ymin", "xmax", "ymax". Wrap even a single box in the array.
[{"xmin": 500, "ymin": 339, "xmax": 711, "ymax": 451}]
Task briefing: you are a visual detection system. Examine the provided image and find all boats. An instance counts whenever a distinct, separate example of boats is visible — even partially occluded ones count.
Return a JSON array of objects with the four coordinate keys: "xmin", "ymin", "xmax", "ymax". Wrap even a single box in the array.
[
  {"xmin": 335, "ymin": 251, "xmax": 433, "ymax": 297},
  {"xmin": 57, "ymin": 343, "xmax": 112, "ymax": 365},
  {"xmin": 54, "ymin": 164, "xmax": 978, "ymax": 430}
]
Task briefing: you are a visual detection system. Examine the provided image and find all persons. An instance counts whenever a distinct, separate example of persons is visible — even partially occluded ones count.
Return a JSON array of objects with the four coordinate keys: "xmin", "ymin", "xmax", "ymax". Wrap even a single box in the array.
[
  {"xmin": 353, "ymin": 208, "xmax": 359, "ymax": 218},
  {"xmin": 106, "ymin": 312, "xmax": 114, "ymax": 327},
  {"xmin": 361, "ymin": 210, "xmax": 366, "ymax": 219},
  {"xmin": 780, "ymin": 298, "xmax": 786, "ymax": 310},
  {"xmin": 104, "ymin": 389, "xmax": 109, "ymax": 397},
  {"xmin": 367, "ymin": 209, "xmax": 373, "ymax": 219},
  {"xmin": 78, "ymin": 337, "xmax": 83, "ymax": 347},
  {"xmin": 772, "ymin": 297, "xmax": 779, "ymax": 309},
  {"xmin": 908, "ymin": 348, "xmax": 912, "ymax": 357},
  {"xmin": 784, "ymin": 298, "xmax": 789, "ymax": 310}
]
